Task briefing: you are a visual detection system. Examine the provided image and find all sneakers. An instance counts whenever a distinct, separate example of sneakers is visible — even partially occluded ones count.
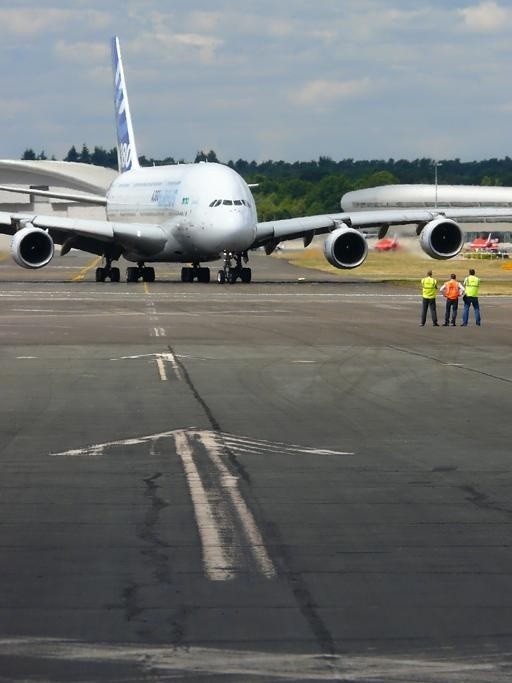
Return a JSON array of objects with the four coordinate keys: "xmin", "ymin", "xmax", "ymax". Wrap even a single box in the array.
[{"xmin": 419, "ymin": 322, "xmax": 482, "ymax": 327}]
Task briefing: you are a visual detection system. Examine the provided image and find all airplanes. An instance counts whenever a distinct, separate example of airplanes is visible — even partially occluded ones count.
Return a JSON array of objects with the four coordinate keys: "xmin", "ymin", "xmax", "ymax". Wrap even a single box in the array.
[{"xmin": 0, "ymin": 35, "xmax": 512, "ymax": 284}]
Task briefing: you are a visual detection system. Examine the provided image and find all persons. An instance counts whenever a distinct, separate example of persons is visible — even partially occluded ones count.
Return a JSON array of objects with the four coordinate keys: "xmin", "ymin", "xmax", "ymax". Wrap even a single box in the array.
[
  {"xmin": 418, "ymin": 269, "xmax": 440, "ymax": 326},
  {"xmin": 439, "ymin": 273, "xmax": 465, "ymax": 326},
  {"xmin": 460, "ymin": 268, "xmax": 480, "ymax": 326}
]
